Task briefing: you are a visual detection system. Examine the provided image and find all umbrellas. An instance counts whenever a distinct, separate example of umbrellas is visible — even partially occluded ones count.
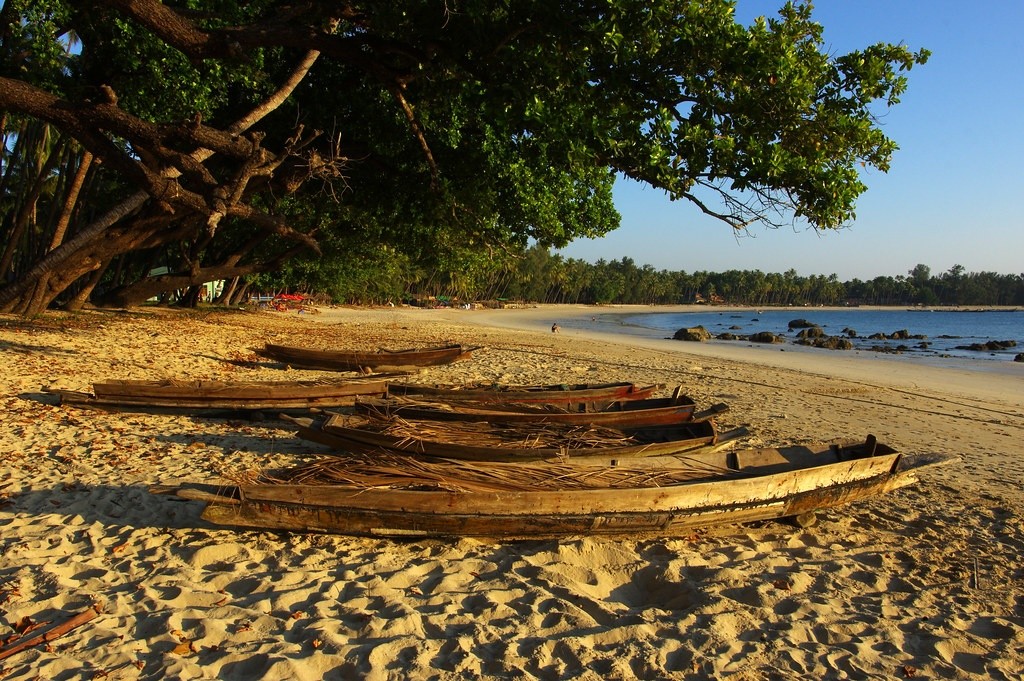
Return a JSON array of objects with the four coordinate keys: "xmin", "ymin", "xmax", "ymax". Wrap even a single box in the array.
[{"xmin": 275, "ymin": 294, "xmax": 305, "ymax": 301}]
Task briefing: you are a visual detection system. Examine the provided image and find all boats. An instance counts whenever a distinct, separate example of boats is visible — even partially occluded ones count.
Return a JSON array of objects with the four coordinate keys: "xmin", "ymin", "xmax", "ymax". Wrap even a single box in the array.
[{"xmin": 40, "ymin": 341, "xmax": 962, "ymax": 543}]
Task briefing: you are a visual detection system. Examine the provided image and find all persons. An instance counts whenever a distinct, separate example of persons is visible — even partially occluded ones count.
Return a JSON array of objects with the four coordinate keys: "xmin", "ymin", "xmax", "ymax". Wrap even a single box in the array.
[
  {"xmin": 552, "ymin": 323, "xmax": 560, "ymax": 333},
  {"xmin": 276, "ymin": 303, "xmax": 282, "ymax": 312}
]
